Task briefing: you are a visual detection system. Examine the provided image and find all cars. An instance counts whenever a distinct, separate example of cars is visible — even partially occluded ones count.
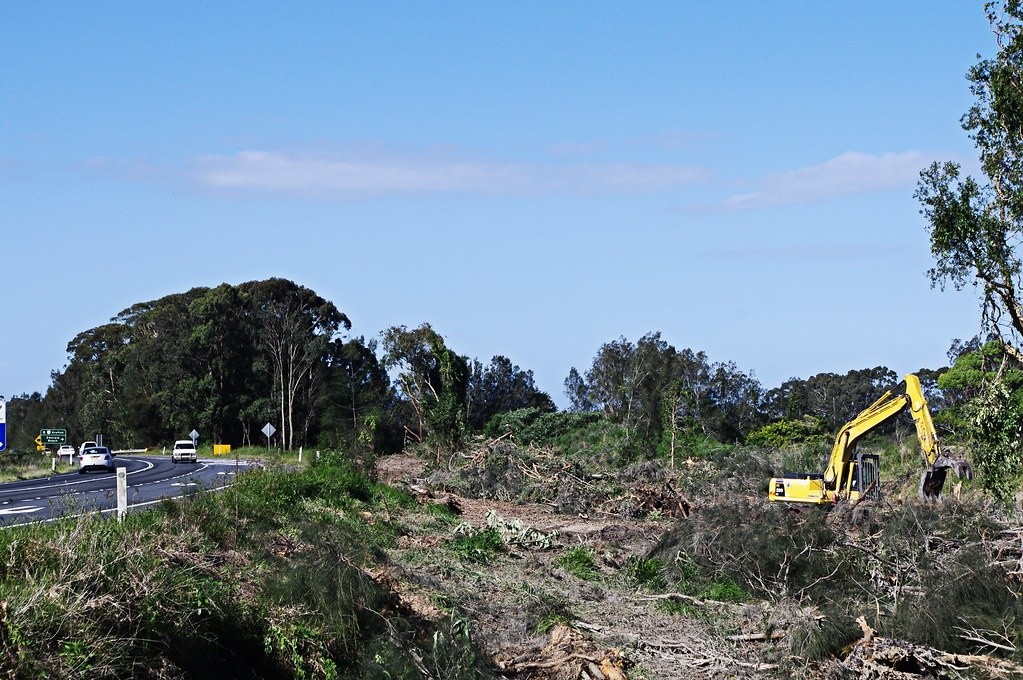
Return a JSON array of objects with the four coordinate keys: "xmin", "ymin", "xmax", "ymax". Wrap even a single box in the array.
[
  {"xmin": 41, "ymin": 447, "xmax": 51, "ymax": 456},
  {"xmin": 57, "ymin": 445, "xmax": 75, "ymax": 457},
  {"xmin": 78, "ymin": 447, "xmax": 116, "ymax": 474}
]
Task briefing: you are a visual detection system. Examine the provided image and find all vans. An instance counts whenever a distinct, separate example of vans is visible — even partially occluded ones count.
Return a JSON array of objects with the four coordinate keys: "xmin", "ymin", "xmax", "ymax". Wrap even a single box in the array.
[{"xmin": 79, "ymin": 441, "xmax": 97, "ymax": 454}]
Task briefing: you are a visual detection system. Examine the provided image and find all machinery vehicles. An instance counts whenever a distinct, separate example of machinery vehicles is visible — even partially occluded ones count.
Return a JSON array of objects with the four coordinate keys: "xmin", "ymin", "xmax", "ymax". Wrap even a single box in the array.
[{"xmin": 768, "ymin": 375, "xmax": 973, "ymax": 517}]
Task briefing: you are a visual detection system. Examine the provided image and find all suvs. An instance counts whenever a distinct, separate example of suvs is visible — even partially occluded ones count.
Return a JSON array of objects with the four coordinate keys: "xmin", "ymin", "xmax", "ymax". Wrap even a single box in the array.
[{"xmin": 172, "ymin": 440, "xmax": 197, "ymax": 463}]
